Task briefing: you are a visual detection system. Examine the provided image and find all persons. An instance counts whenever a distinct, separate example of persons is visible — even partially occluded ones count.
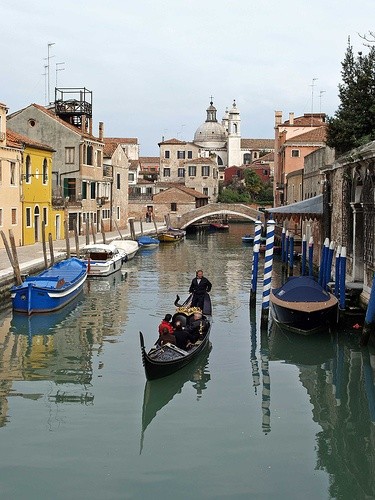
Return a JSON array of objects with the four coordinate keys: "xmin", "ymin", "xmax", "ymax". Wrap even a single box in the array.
[
  {"xmin": 159, "ymin": 327, "xmax": 176, "ymax": 345},
  {"xmin": 190, "ymin": 313, "xmax": 206, "ymax": 334},
  {"xmin": 188, "ymin": 269, "xmax": 212, "ymax": 312},
  {"xmin": 172, "ymin": 321, "xmax": 198, "ymax": 351},
  {"xmin": 158, "ymin": 314, "xmax": 174, "ymax": 336}
]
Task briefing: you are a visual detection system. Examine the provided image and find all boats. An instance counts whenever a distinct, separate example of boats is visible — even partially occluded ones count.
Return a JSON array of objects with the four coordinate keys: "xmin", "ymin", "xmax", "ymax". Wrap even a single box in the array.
[
  {"xmin": 241, "ymin": 233, "xmax": 254, "ymax": 243},
  {"xmin": 268, "ymin": 268, "xmax": 340, "ymax": 336},
  {"xmin": 210, "ymin": 221, "xmax": 231, "ymax": 233},
  {"xmin": 10, "ymin": 257, "xmax": 86, "ymax": 317},
  {"xmin": 138, "ymin": 235, "xmax": 159, "ymax": 250},
  {"xmin": 139, "ymin": 291, "xmax": 214, "ymax": 373},
  {"xmin": 108, "ymin": 240, "xmax": 140, "ymax": 261},
  {"xmin": 75, "ymin": 244, "xmax": 122, "ymax": 278},
  {"xmin": 159, "ymin": 229, "xmax": 188, "ymax": 243}
]
